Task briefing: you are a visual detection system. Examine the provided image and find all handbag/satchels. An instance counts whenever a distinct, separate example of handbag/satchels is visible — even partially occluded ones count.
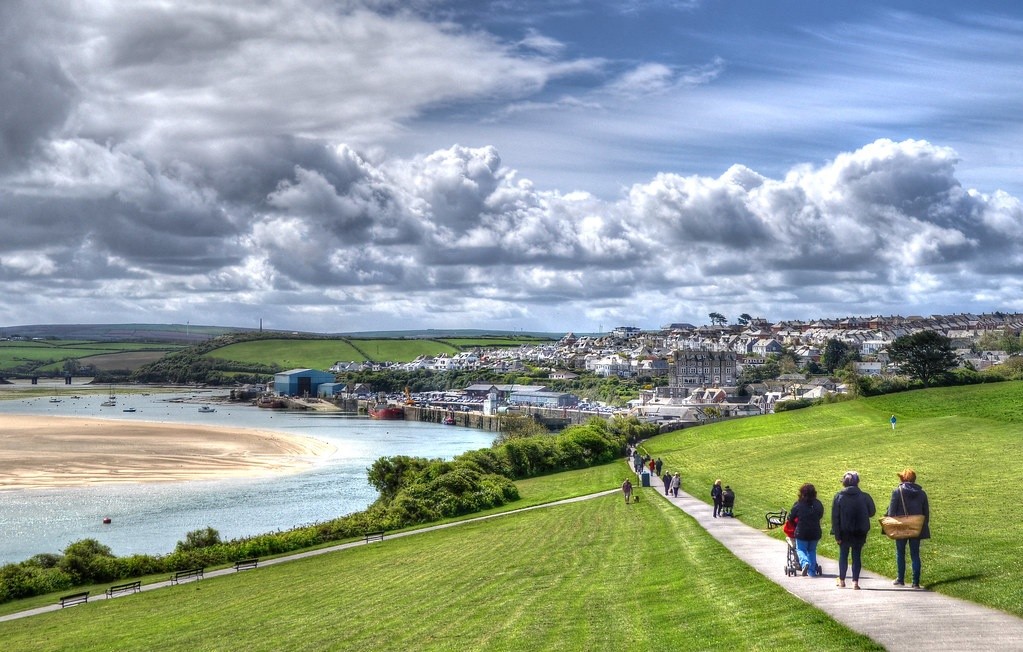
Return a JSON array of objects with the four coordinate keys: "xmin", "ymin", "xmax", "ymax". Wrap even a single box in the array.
[
  {"xmin": 879, "ymin": 515, "xmax": 925, "ymax": 538},
  {"xmin": 669, "ymin": 488, "xmax": 673, "ymax": 493}
]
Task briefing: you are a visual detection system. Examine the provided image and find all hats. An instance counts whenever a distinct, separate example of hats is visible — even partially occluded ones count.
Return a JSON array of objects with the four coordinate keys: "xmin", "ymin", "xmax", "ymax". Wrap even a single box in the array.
[
  {"xmin": 898, "ymin": 469, "xmax": 915, "ymax": 482},
  {"xmin": 725, "ymin": 486, "xmax": 729, "ymax": 488},
  {"xmin": 675, "ymin": 472, "xmax": 678, "ymax": 474},
  {"xmin": 666, "ymin": 471, "xmax": 668, "ymax": 473}
]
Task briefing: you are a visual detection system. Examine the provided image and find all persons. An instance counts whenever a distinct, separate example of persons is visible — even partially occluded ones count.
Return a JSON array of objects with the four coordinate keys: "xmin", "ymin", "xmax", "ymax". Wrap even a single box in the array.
[
  {"xmin": 632, "ymin": 434, "xmax": 637, "ymax": 447},
  {"xmin": 830, "ymin": 471, "xmax": 877, "ymax": 590},
  {"xmin": 655, "ymin": 458, "xmax": 663, "ymax": 476},
  {"xmin": 644, "ymin": 454, "xmax": 650, "ymax": 462},
  {"xmin": 633, "ymin": 453, "xmax": 645, "ymax": 474},
  {"xmin": 881, "ymin": 468, "xmax": 931, "ymax": 589},
  {"xmin": 622, "ymin": 478, "xmax": 632, "ymax": 501},
  {"xmin": 633, "ymin": 450, "xmax": 637, "ymax": 458},
  {"xmin": 890, "ymin": 415, "xmax": 896, "ymax": 430},
  {"xmin": 722, "ymin": 485, "xmax": 734, "ymax": 513},
  {"xmin": 627, "ymin": 447, "xmax": 632, "ymax": 457},
  {"xmin": 648, "ymin": 459, "xmax": 655, "ymax": 476},
  {"xmin": 663, "ymin": 471, "xmax": 672, "ymax": 495},
  {"xmin": 787, "ymin": 483, "xmax": 824, "ymax": 577},
  {"xmin": 670, "ymin": 472, "xmax": 680, "ymax": 498},
  {"xmin": 711, "ymin": 479, "xmax": 723, "ymax": 518}
]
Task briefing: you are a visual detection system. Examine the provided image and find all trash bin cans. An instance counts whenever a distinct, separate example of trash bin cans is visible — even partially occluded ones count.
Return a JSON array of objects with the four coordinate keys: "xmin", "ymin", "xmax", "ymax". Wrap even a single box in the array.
[{"xmin": 642, "ymin": 471, "xmax": 650, "ymax": 487}]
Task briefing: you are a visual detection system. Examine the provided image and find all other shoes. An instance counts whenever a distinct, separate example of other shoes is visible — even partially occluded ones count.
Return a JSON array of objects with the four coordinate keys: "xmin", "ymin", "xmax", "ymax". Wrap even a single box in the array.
[
  {"xmin": 802, "ymin": 563, "xmax": 809, "ymax": 576},
  {"xmin": 854, "ymin": 585, "xmax": 860, "ymax": 590},
  {"xmin": 893, "ymin": 578, "xmax": 904, "ymax": 587},
  {"xmin": 912, "ymin": 583, "xmax": 919, "ymax": 589}
]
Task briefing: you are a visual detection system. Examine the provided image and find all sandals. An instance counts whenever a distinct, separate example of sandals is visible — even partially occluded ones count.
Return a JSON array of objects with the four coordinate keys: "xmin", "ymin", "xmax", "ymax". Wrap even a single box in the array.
[{"xmin": 836, "ymin": 580, "xmax": 844, "ymax": 587}]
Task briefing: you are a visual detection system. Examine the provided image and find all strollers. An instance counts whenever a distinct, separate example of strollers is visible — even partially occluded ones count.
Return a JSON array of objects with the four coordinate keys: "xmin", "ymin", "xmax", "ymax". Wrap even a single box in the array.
[{"xmin": 784, "ymin": 514, "xmax": 823, "ymax": 577}]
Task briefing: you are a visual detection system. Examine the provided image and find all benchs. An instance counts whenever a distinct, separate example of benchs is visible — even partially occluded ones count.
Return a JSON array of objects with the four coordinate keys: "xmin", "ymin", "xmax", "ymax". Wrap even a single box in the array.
[
  {"xmin": 361, "ymin": 530, "xmax": 386, "ymax": 542},
  {"xmin": 58, "ymin": 591, "xmax": 89, "ymax": 608},
  {"xmin": 232, "ymin": 558, "xmax": 258, "ymax": 572},
  {"xmin": 766, "ymin": 506, "xmax": 787, "ymax": 529},
  {"xmin": 105, "ymin": 580, "xmax": 141, "ymax": 600},
  {"xmin": 170, "ymin": 567, "xmax": 204, "ymax": 586}
]
